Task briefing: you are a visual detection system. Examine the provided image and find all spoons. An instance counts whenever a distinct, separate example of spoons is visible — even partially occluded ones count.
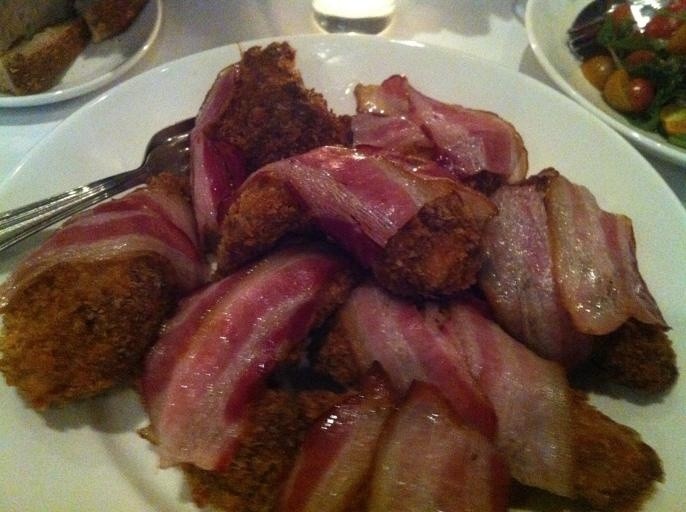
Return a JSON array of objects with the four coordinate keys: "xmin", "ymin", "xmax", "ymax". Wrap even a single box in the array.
[{"xmin": 0, "ymin": 115, "xmax": 197, "ymax": 256}]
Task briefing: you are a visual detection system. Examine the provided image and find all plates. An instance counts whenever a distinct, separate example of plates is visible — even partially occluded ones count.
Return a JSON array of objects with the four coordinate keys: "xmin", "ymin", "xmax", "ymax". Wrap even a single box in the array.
[
  {"xmin": 0, "ymin": 1, "xmax": 165, "ymax": 109},
  {"xmin": 0, "ymin": 31, "xmax": 686, "ymax": 512},
  {"xmin": 523, "ymin": 0, "xmax": 686, "ymax": 171}
]
